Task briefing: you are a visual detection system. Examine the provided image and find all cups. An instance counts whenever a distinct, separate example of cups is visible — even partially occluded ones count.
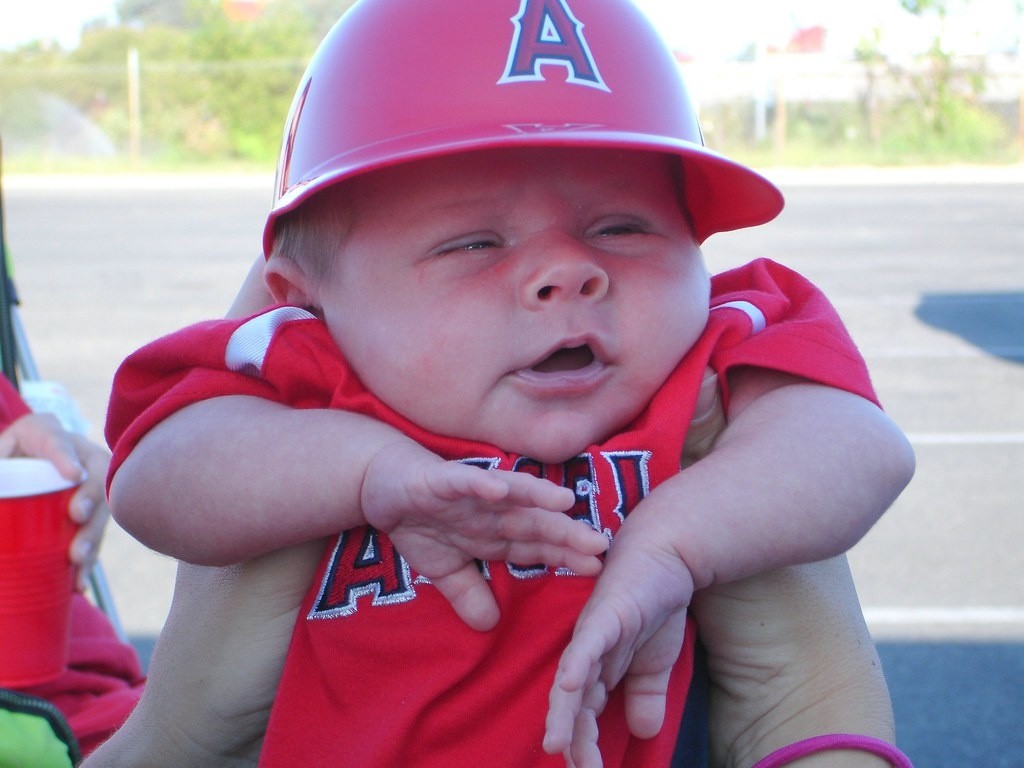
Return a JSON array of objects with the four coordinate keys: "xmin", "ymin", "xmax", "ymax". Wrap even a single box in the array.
[{"xmin": 0, "ymin": 456, "xmax": 87, "ymax": 686}]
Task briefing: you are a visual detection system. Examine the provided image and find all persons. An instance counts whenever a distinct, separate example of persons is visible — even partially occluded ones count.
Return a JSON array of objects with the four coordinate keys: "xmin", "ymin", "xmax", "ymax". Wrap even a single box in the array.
[
  {"xmin": 0, "ymin": 316, "xmax": 113, "ymax": 596},
  {"xmin": 74, "ymin": 0, "xmax": 918, "ymax": 768}
]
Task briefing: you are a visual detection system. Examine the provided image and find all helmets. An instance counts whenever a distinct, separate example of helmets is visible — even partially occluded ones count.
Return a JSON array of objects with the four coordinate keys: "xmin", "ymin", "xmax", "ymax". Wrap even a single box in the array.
[{"xmin": 262, "ymin": 1, "xmax": 786, "ymax": 261}]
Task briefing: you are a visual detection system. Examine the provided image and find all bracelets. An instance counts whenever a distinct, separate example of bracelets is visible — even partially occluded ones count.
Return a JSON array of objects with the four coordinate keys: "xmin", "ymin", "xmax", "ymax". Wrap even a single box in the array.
[{"xmin": 750, "ymin": 731, "xmax": 912, "ymax": 768}]
